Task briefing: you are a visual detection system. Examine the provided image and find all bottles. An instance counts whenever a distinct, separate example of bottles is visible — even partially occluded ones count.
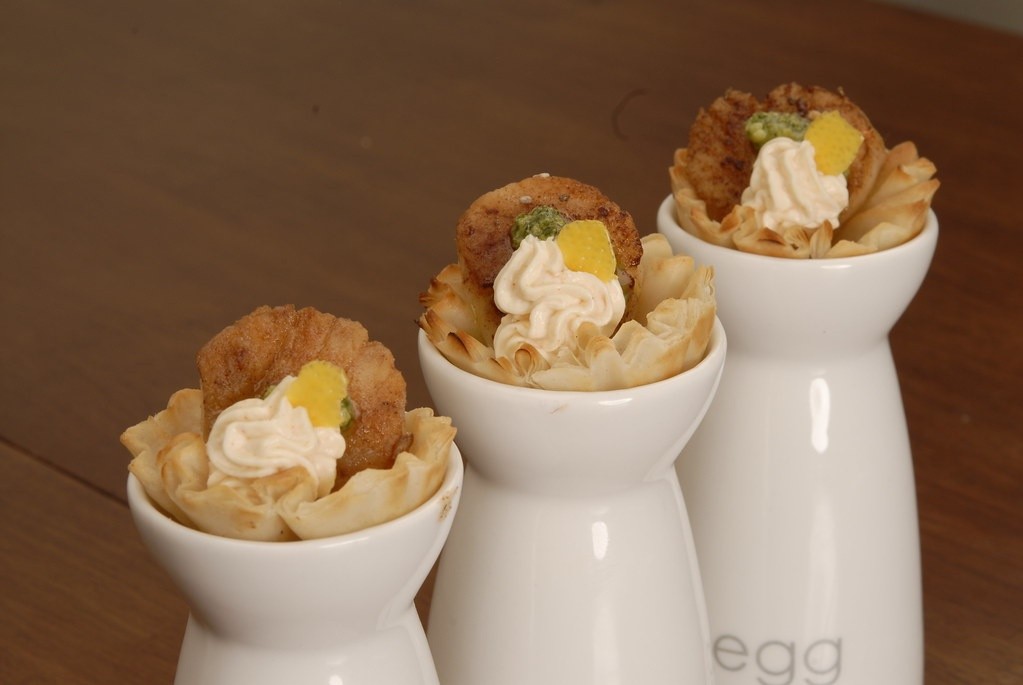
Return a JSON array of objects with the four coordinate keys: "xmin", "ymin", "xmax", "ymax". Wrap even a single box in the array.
[
  {"xmin": 419, "ymin": 313, "xmax": 728, "ymax": 682},
  {"xmin": 126, "ymin": 412, "xmax": 465, "ymax": 683},
  {"xmin": 657, "ymin": 193, "xmax": 941, "ymax": 685}
]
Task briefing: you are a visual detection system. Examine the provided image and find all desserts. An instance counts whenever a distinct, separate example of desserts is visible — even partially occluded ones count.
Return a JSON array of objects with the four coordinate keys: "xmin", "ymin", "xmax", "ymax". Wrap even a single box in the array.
[{"xmin": 116, "ymin": 83, "xmax": 940, "ymax": 545}]
